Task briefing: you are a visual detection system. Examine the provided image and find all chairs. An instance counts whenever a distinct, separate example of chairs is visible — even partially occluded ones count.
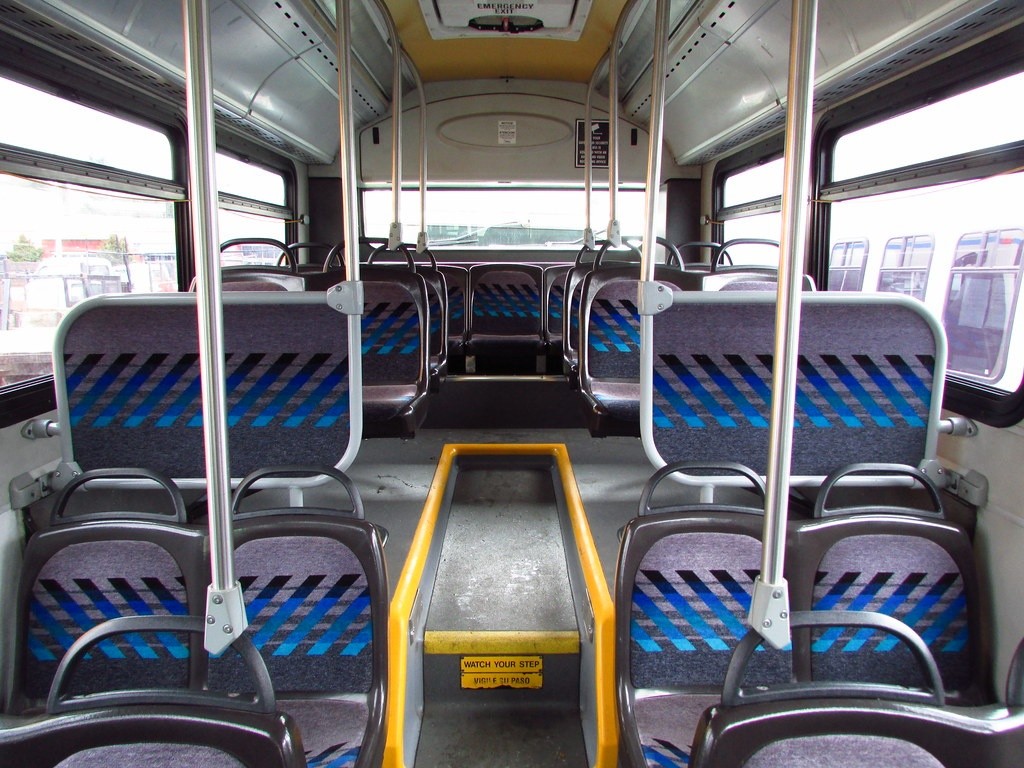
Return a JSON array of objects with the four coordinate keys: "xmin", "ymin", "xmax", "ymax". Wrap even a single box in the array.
[
  {"xmin": 189, "ymin": 237, "xmax": 989, "ymax": 509},
  {"xmin": 0, "ymin": 461, "xmax": 395, "ymax": 768},
  {"xmin": 614, "ymin": 462, "xmax": 1024, "ymax": 768}
]
